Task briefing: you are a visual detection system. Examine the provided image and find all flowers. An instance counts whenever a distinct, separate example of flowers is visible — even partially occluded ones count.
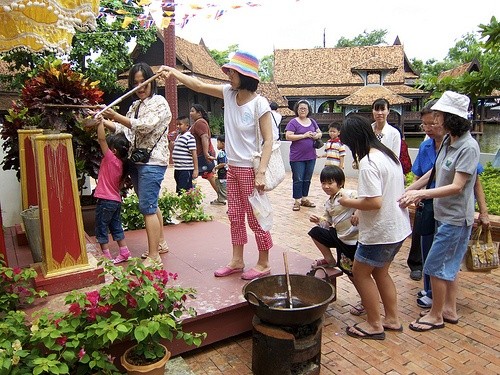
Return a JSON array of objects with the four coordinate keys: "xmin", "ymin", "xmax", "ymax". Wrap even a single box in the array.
[{"xmin": 0, "ymin": 254, "xmax": 207, "ymax": 375}]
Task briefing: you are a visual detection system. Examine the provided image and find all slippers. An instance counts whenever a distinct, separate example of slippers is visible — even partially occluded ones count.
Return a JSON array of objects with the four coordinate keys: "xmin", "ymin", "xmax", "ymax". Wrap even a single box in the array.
[
  {"xmin": 311, "ymin": 258, "xmax": 337, "ymax": 268},
  {"xmin": 409, "ymin": 317, "xmax": 445, "ymax": 332},
  {"xmin": 346, "ymin": 323, "xmax": 386, "ymax": 340},
  {"xmin": 420, "ymin": 309, "xmax": 458, "ymax": 324},
  {"xmin": 214, "ymin": 264, "xmax": 243, "ymax": 277},
  {"xmin": 241, "ymin": 267, "xmax": 272, "ymax": 280},
  {"xmin": 349, "ymin": 302, "xmax": 367, "ymax": 316},
  {"xmin": 383, "ymin": 325, "xmax": 404, "ymax": 332}
]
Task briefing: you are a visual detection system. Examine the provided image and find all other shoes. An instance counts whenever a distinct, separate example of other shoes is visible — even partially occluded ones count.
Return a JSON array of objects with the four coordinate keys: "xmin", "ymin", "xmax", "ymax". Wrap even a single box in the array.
[
  {"xmin": 210, "ymin": 198, "xmax": 226, "ymax": 206},
  {"xmin": 410, "ymin": 270, "xmax": 423, "ymax": 281},
  {"xmin": 416, "ymin": 290, "xmax": 434, "ymax": 308}
]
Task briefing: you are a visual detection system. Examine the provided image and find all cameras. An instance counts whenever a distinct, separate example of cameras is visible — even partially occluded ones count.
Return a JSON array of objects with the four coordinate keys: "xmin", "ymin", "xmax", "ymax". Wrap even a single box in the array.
[{"xmin": 130, "ymin": 147, "xmax": 151, "ymax": 163}]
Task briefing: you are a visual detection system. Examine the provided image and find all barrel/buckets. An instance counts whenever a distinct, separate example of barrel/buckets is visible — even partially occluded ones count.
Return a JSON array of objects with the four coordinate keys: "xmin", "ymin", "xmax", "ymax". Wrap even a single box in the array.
[{"xmin": 20, "ymin": 206, "xmax": 44, "ymax": 263}]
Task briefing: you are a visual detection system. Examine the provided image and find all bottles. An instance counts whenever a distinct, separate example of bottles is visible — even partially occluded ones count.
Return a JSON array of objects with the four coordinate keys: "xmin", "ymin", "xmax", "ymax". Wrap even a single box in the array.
[{"xmin": 168, "ymin": 129, "xmax": 179, "ymax": 140}]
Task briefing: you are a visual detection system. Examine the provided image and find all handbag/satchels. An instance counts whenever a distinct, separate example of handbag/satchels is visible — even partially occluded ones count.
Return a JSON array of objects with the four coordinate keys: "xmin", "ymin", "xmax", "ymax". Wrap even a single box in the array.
[
  {"xmin": 310, "ymin": 118, "xmax": 325, "ymax": 149},
  {"xmin": 465, "ymin": 225, "xmax": 499, "ymax": 274},
  {"xmin": 415, "ymin": 200, "xmax": 434, "ymax": 236},
  {"xmin": 248, "ymin": 186, "xmax": 274, "ymax": 232},
  {"xmin": 399, "ymin": 139, "xmax": 412, "ymax": 175},
  {"xmin": 252, "ymin": 97, "xmax": 286, "ymax": 192}
]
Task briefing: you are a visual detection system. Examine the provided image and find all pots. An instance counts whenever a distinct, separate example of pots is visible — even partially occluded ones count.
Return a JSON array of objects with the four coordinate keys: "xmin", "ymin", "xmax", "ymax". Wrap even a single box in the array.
[{"xmin": 242, "ymin": 265, "xmax": 336, "ymax": 328}]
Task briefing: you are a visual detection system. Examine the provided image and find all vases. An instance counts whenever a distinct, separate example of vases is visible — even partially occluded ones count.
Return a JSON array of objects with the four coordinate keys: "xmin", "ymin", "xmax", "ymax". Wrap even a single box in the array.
[{"xmin": 121, "ymin": 341, "xmax": 170, "ymax": 375}]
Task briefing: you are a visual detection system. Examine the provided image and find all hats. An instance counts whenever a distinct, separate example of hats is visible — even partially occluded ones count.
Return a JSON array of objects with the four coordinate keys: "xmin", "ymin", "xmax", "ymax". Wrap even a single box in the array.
[
  {"xmin": 430, "ymin": 91, "xmax": 470, "ymax": 119},
  {"xmin": 222, "ymin": 50, "xmax": 261, "ymax": 83}
]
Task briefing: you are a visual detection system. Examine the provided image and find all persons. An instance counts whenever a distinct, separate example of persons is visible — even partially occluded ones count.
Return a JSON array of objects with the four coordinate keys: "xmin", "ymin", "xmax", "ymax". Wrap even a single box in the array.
[
  {"xmin": 397, "ymin": 90, "xmax": 490, "ymax": 332},
  {"xmin": 169, "ymin": 116, "xmax": 199, "ymax": 195},
  {"xmin": 338, "ymin": 116, "xmax": 414, "ymax": 340},
  {"xmin": 318, "ymin": 121, "xmax": 346, "ymax": 170},
  {"xmin": 284, "ymin": 98, "xmax": 322, "ymax": 210},
  {"xmin": 158, "ymin": 51, "xmax": 273, "ymax": 281},
  {"xmin": 188, "ymin": 104, "xmax": 218, "ymax": 193},
  {"xmin": 351, "ymin": 98, "xmax": 411, "ymax": 175},
  {"xmin": 207, "ymin": 135, "xmax": 228, "ymax": 205},
  {"xmin": 93, "ymin": 62, "xmax": 171, "ymax": 275},
  {"xmin": 269, "ymin": 102, "xmax": 283, "ymax": 139},
  {"xmin": 306, "ymin": 165, "xmax": 367, "ymax": 315},
  {"xmin": 93, "ymin": 112, "xmax": 134, "ymax": 264}
]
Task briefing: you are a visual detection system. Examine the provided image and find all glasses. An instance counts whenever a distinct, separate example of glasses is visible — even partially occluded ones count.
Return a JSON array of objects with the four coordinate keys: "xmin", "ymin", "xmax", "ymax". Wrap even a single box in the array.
[
  {"xmin": 419, "ymin": 123, "xmax": 438, "ymax": 130},
  {"xmin": 226, "ymin": 70, "xmax": 237, "ymax": 76}
]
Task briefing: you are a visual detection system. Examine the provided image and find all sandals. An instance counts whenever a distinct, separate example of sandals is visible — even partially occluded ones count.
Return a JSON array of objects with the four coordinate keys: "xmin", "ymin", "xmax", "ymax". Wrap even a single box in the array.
[
  {"xmin": 292, "ymin": 199, "xmax": 316, "ymax": 211},
  {"xmin": 130, "ymin": 256, "xmax": 164, "ymax": 276},
  {"xmin": 141, "ymin": 241, "xmax": 169, "ymax": 258},
  {"xmin": 113, "ymin": 251, "xmax": 132, "ymax": 263}
]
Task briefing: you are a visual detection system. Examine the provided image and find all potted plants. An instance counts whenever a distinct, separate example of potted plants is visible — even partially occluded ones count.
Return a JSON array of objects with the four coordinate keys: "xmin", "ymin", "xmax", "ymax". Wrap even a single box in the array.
[{"xmin": 0, "ymin": 58, "xmax": 120, "ymax": 236}]
